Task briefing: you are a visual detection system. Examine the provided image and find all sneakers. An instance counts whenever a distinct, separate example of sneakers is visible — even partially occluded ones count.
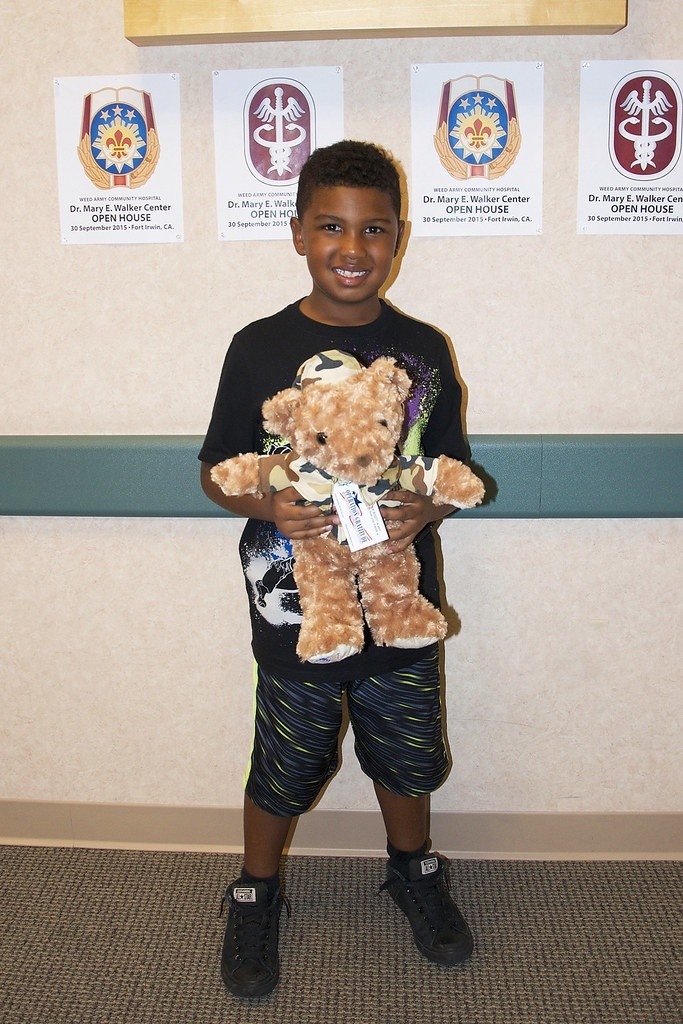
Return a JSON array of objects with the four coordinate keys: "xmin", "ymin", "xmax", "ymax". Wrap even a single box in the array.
[
  {"xmin": 384, "ymin": 853, "xmax": 475, "ymax": 969},
  {"xmin": 220, "ymin": 880, "xmax": 284, "ymax": 1001}
]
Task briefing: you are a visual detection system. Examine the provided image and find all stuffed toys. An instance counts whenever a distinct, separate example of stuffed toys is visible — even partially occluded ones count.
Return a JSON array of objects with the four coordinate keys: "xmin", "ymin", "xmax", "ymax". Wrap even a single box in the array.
[{"xmin": 208, "ymin": 350, "xmax": 485, "ymax": 664}]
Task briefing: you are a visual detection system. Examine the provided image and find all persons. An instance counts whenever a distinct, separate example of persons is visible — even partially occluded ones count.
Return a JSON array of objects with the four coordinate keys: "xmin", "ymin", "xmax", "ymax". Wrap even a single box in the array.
[{"xmin": 198, "ymin": 138, "xmax": 498, "ymax": 999}]
